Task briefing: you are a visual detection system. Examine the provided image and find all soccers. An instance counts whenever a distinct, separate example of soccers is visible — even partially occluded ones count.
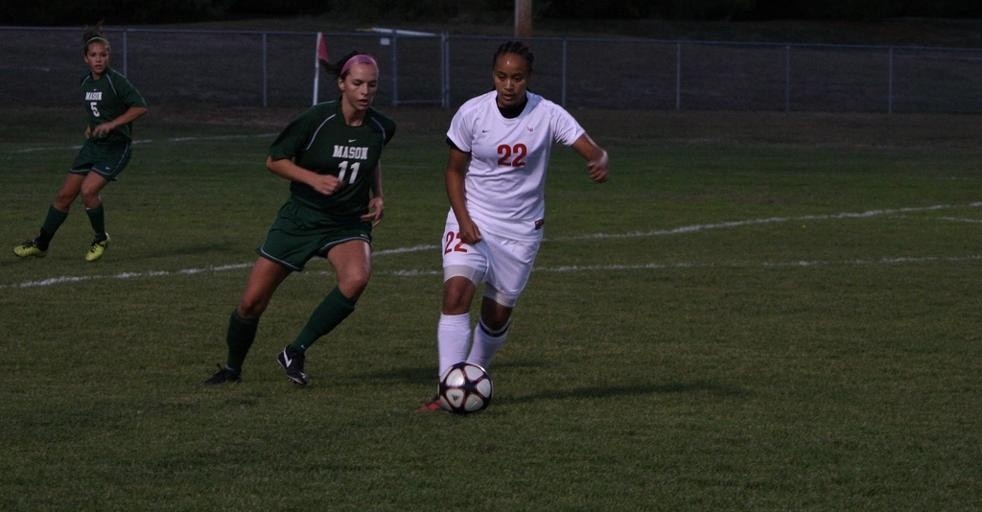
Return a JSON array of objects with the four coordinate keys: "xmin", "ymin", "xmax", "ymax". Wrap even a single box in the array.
[{"xmin": 436, "ymin": 362, "xmax": 494, "ymax": 413}]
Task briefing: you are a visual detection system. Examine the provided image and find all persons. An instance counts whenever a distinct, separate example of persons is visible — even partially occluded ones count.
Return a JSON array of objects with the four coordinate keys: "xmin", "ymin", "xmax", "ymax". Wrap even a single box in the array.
[
  {"xmin": 202, "ymin": 51, "xmax": 397, "ymax": 389},
  {"xmin": 11, "ymin": 34, "xmax": 149, "ymax": 263},
  {"xmin": 435, "ymin": 40, "xmax": 609, "ymax": 397}
]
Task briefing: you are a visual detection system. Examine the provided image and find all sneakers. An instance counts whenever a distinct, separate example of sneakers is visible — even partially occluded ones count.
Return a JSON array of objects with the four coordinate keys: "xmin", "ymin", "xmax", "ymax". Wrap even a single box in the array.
[
  {"xmin": 203, "ymin": 368, "xmax": 243, "ymax": 385},
  {"xmin": 277, "ymin": 344, "xmax": 309, "ymax": 385},
  {"xmin": 13, "ymin": 240, "xmax": 48, "ymax": 258},
  {"xmin": 86, "ymin": 232, "xmax": 111, "ymax": 261}
]
